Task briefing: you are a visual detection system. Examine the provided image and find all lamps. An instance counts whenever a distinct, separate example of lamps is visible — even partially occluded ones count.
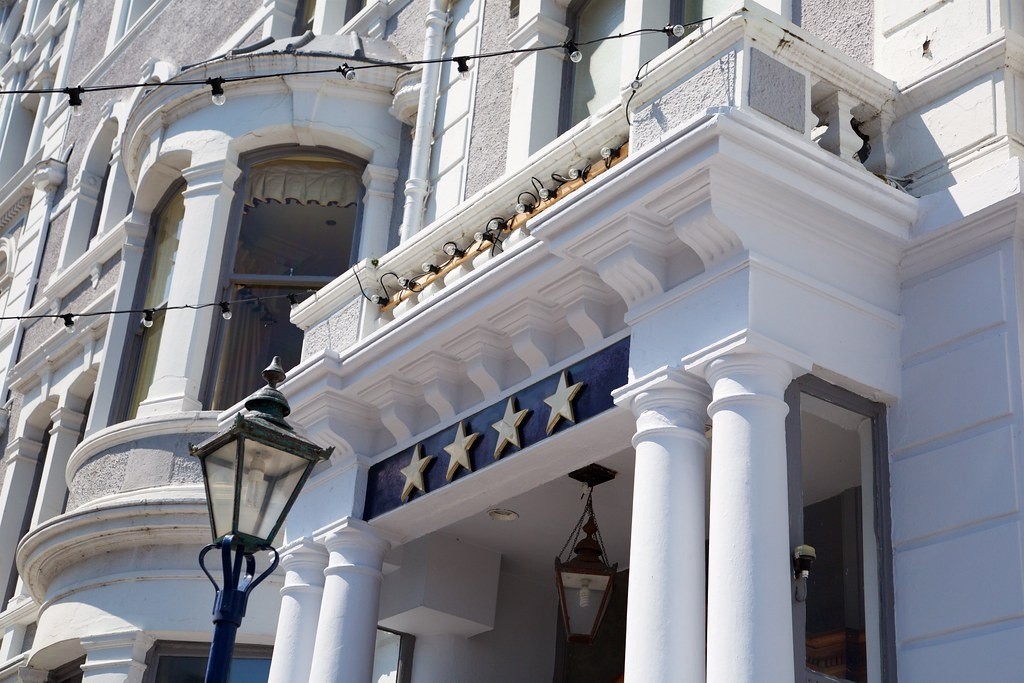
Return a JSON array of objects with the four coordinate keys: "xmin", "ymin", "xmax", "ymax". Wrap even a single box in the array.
[{"xmin": 553, "ymin": 464, "xmax": 619, "ymax": 645}]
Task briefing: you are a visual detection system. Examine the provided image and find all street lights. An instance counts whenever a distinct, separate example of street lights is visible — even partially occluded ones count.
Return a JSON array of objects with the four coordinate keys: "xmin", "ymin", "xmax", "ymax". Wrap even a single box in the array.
[{"xmin": 187, "ymin": 355, "xmax": 336, "ymax": 683}]
